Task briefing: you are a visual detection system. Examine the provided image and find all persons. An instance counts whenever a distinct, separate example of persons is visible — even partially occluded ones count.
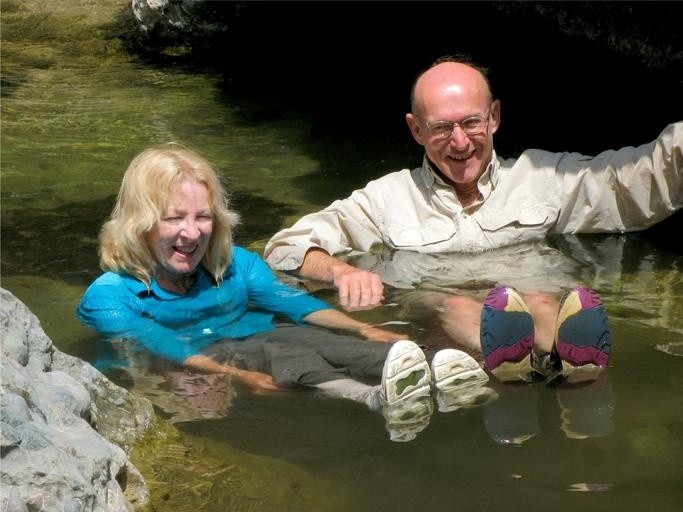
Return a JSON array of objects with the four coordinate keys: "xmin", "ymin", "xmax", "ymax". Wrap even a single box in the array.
[
  {"xmin": 74, "ymin": 139, "xmax": 492, "ymax": 446},
  {"xmin": 261, "ymin": 53, "xmax": 683, "ymax": 391}
]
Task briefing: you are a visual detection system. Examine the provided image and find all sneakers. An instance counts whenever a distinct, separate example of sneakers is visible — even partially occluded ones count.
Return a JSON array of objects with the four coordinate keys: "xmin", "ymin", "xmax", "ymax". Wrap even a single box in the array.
[
  {"xmin": 542, "ymin": 285, "xmax": 613, "ymax": 389},
  {"xmin": 429, "ymin": 347, "xmax": 490, "ymax": 391},
  {"xmin": 380, "ymin": 384, "xmax": 617, "ymax": 447},
  {"xmin": 366, "ymin": 338, "xmax": 432, "ymax": 407},
  {"xmin": 478, "ymin": 283, "xmax": 545, "ymax": 386}
]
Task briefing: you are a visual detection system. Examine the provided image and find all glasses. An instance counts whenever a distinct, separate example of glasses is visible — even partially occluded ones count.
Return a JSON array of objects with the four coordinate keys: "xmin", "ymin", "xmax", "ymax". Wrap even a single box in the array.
[{"xmin": 414, "ymin": 108, "xmax": 493, "ymax": 139}]
